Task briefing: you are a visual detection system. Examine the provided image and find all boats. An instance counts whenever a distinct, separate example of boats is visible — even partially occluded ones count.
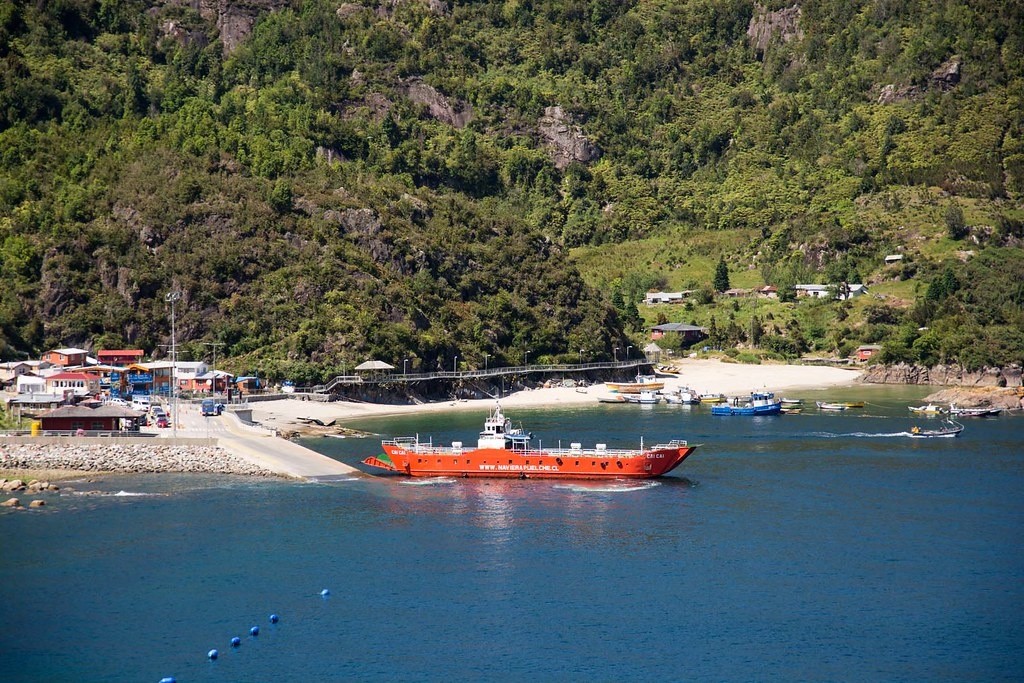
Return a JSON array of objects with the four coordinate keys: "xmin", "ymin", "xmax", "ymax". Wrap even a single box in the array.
[
  {"xmin": 604, "ymin": 365, "xmax": 664, "ymax": 394},
  {"xmin": 907, "ymin": 403, "xmax": 951, "ymax": 419},
  {"xmin": 911, "ymin": 417, "xmax": 964, "ymax": 437},
  {"xmin": 598, "ymin": 387, "xmax": 701, "ymax": 405},
  {"xmin": 698, "ymin": 391, "xmax": 727, "ymax": 403},
  {"xmin": 712, "ymin": 386, "xmax": 781, "ymax": 416},
  {"xmin": 948, "ymin": 403, "xmax": 1002, "ymax": 417},
  {"xmin": 781, "ymin": 409, "xmax": 803, "ymax": 414},
  {"xmin": 815, "ymin": 401, "xmax": 849, "ymax": 410},
  {"xmin": 779, "ymin": 397, "xmax": 805, "ymax": 404},
  {"xmin": 358, "ymin": 401, "xmax": 704, "ymax": 482},
  {"xmin": 656, "ymin": 365, "xmax": 683, "ymax": 373},
  {"xmin": 831, "ymin": 401, "xmax": 864, "ymax": 408}
]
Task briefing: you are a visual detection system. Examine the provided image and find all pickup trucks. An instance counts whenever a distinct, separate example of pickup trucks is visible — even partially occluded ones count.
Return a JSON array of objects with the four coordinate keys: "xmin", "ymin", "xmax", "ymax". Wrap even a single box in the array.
[{"xmin": 105, "ymin": 399, "xmax": 130, "ymax": 406}]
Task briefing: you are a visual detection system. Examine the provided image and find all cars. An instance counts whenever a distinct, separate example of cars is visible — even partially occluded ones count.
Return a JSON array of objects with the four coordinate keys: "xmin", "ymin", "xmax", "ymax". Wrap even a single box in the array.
[{"xmin": 133, "ymin": 400, "xmax": 172, "ymax": 428}]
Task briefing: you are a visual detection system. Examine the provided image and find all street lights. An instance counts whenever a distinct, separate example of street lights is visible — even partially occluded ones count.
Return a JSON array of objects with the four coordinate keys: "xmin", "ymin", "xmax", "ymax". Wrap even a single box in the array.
[
  {"xmin": 166, "ymin": 351, "xmax": 191, "ymax": 427},
  {"xmin": 404, "ymin": 359, "xmax": 409, "ymax": 374},
  {"xmin": 614, "ymin": 348, "xmax": 619, "ymax": 362},
  {"xmin": 486, "ymin": 355, "xmax": 491, "ymax": 373},
  {"xmin": 579, "ymin": 350, "xmax": 584, "ymax": 364},
  {"xmin": 525, "ymin": 351, "xmax": 531, "ymax": 370},
  {"xmin": 627, "ymin": 346, "xmax": 633, "ymax": 361},
  {"xmin": 157, "ymin": 344, "xmax": 182, "ymax": 422},
  {"xmin": 454, "ymin": 356, "xmax": 457, "ymax": 376},
  {"xmin": 203, "ymin": 342, "xmax": 226, "ymax": 406}
]
